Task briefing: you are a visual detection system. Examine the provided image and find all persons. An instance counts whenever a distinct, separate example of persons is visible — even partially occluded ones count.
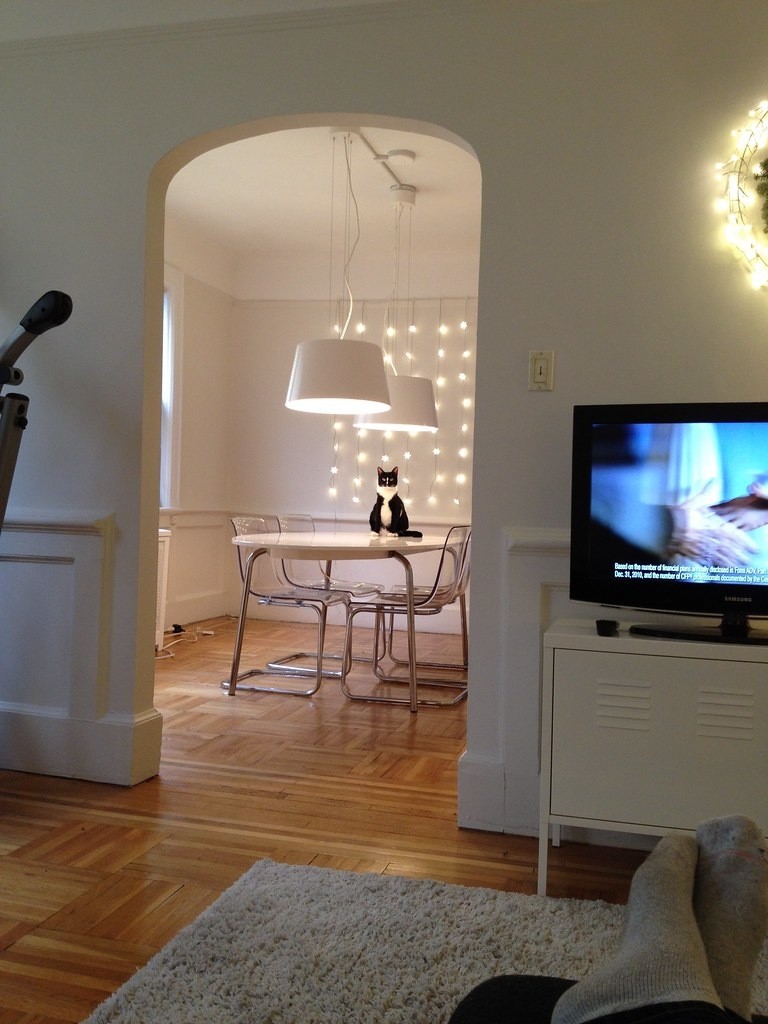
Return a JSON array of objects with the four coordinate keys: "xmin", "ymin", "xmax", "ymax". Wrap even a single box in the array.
[
  {"xmin": 549, "ymin": 813, "xmax": 768, "ymax": 1024},
  {"xmin": 593, "ymin": 423, "xmax": 768, "ymax": 568}
]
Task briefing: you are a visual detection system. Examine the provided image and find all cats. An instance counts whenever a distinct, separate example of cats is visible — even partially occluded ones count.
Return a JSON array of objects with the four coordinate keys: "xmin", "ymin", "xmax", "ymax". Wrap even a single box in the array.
[{"xmin": 369, "ymin": 467, "xmax": 423, "ymax": 538}]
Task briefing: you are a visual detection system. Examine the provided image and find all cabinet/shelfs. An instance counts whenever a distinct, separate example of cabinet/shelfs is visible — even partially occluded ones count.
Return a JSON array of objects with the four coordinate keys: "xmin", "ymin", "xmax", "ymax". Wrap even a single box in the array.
[{"xmin": 537, "ymin": 615, "xmax": 766, "ymax": 897}]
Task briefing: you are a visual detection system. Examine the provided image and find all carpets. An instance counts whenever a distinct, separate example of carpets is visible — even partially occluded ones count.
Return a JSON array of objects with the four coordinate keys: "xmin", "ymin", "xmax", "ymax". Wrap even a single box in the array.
[{"xmin": 74, "ymin": 859, "xmax": 767, "ymax": 1024}]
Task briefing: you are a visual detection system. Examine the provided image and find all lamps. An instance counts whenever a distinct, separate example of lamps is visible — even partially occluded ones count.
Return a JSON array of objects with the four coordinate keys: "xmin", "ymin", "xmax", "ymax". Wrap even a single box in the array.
[{"xmin": 283, "ymin": 123, "xmax": 442, "ymax": 430}]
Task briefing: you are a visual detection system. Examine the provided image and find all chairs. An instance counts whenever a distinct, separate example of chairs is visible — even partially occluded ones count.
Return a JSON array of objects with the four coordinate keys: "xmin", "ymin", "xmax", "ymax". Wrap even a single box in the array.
[{"xmin": 231, "ymin": 515, "xmax": 476, "ymax": 708}]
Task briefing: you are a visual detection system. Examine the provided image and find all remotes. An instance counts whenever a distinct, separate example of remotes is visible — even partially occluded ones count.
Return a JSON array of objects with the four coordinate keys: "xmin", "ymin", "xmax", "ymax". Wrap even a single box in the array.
[{"xmin": 595, "ymin": 619, "xmax": 620, "ymax": 637}]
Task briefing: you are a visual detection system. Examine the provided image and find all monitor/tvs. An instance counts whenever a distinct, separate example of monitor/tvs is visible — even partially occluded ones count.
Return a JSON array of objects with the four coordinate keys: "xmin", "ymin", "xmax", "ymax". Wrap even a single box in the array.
[{"xmin": 569, "ymin": 402, "xmax": 768, "ymax": 648}]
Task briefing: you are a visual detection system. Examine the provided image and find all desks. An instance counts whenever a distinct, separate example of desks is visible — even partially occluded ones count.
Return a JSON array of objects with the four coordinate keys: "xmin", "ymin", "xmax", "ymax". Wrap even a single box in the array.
[{"xmin": 234, "ymin": 530, "xmax": 469, "ymax": 713}]
